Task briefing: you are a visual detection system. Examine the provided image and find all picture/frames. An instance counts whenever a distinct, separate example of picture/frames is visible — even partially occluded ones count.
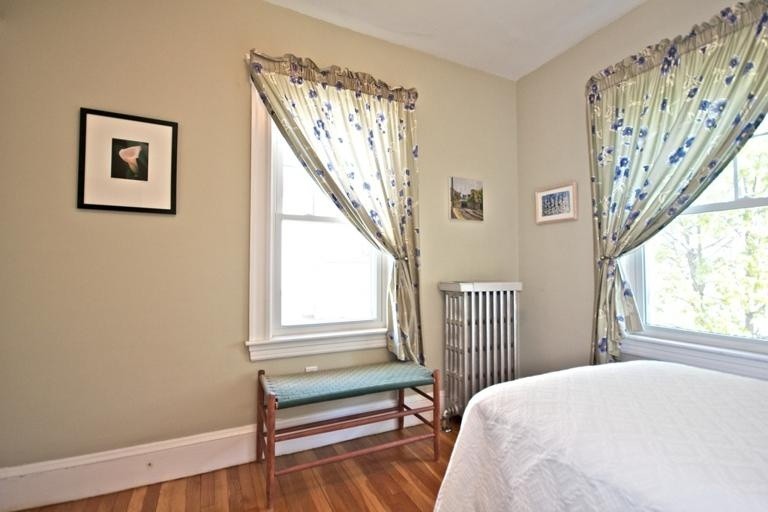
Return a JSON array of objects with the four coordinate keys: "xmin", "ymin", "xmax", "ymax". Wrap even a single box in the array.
[
  {"xmin": 533, "ymin": 181, "xmax": 576, "ymax": 225},
  {"xmin": 451, "ymin": 176, "xmax": 485, "ymax": 222},
  {"xmin": 76, "ymin": 107, "xmax": 178, "ymax": 214}
]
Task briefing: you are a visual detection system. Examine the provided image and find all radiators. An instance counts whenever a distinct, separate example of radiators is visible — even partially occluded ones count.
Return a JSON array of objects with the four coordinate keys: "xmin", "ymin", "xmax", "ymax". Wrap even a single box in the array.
[{"xmin": 438, "ymin": 281, "xmax": 523, "ymax": 431}]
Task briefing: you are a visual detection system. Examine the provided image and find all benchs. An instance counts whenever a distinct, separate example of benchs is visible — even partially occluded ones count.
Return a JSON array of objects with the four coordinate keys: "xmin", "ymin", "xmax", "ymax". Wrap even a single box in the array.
[{"xmin": 255, "ymin": 369, "xmax": 440, "ymax": 508}]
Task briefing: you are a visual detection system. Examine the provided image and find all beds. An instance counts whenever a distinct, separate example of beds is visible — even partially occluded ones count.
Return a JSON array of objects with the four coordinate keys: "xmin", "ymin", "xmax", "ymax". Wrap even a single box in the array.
[{"xmin": 433, "ymin": 358, "xmax": 768, "ymax": 511}]
